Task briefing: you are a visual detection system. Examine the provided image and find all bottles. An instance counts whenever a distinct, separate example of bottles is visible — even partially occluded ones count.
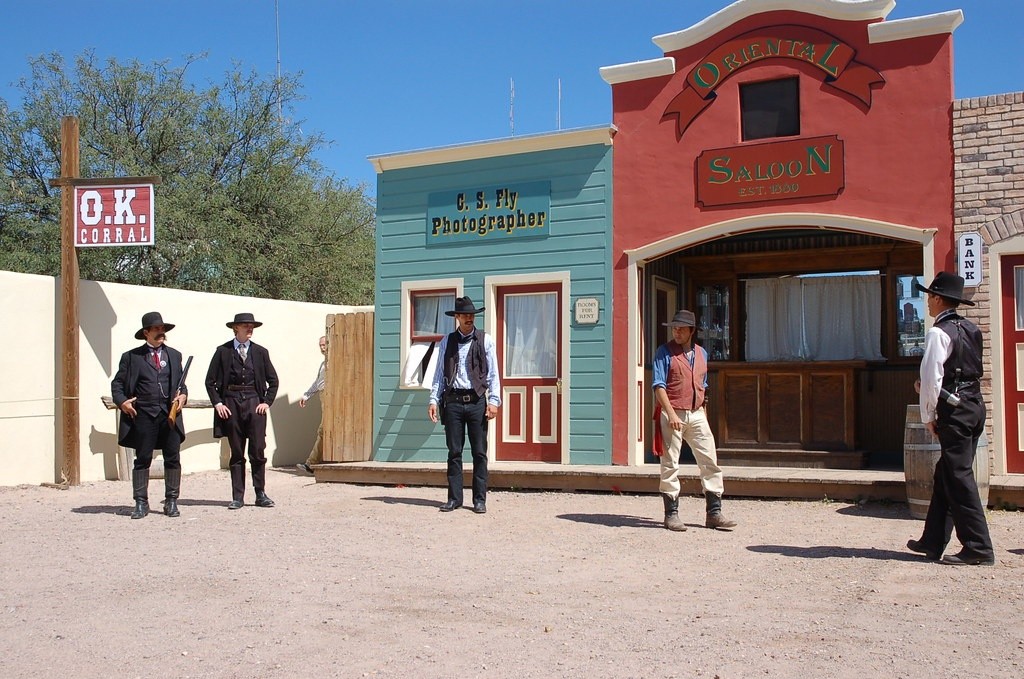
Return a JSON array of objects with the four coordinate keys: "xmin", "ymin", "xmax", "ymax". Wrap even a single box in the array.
[
  {"xmin": 896, "ymin": 276, "xmax": 924, "ymax": 356},
  {"xmin": 695, "ymin": 285, "xmax": 729, "ymax": 360}
]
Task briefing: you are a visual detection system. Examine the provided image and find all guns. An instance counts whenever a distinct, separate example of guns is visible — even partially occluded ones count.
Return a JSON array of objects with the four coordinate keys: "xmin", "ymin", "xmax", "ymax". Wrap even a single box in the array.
[{"xmin": 168, "ymin": 355, "xmax": 193, "ymax": 431}]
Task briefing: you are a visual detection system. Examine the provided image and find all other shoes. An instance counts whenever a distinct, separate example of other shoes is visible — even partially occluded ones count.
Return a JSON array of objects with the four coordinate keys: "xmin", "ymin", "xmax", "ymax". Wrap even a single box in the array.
[{"xmin": 296, "ymin": 463, "xmax": 315, "ymax": 475}]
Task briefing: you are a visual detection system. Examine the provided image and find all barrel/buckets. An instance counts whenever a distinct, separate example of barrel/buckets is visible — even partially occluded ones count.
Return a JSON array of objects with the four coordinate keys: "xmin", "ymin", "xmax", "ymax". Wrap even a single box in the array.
[{"xmin": 904, "ymin": 405, "xmax": 989, "ymax": 519}]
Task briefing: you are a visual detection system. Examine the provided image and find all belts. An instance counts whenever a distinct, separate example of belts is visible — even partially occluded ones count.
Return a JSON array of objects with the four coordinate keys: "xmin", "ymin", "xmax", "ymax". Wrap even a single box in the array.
[
  {"xmin": 227, "ymin": 385, "xmax": 256, "ymax": 391},
  {"xmin": 450, "ymin": 393, "xmax": 479, "ymax": 403}
]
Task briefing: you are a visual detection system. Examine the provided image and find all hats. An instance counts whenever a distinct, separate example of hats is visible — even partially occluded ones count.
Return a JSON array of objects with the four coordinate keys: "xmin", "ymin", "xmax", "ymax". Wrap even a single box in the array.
[
  {"xmin": 226, "ymin": 313, "xmax": 263, "ymax": 328},
  {"xmin": 135, "ymin": 312, "xmax": 175, "ymax": 339},
  {"xmin": 444, "ymin": 296, "xmax": 486, "ymax": 316},
  {"xmin": 662, "ymin": 309, "xmax": 698, "ymax": 329},
  {"xmin": 915, "ymin": 271, "xmax": 975, "ymax": 306}
]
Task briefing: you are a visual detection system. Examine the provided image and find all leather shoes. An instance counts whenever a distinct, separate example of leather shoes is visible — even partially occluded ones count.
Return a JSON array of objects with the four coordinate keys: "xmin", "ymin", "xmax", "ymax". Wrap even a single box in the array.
[
  {"xmin": 255, "ymin": 492, "xmax": 275, "ymax": 506},
  {"xmin": 906, "ymin": 539, "xmax": 943, "ymax": 561},
  {"xmin": 228, "ymin": 499, "xmax": 245, "ymax": 508},
  {"xmin": 942, "ymin": 551, "xmax": 995, "ymax": 566},
  {"xmin": 473, "ymin": 502, "xmax": 487, "ymax": 513},
  {"xmin": 439, "ymin": 499, "xmax": 462, "ymax": 512}
]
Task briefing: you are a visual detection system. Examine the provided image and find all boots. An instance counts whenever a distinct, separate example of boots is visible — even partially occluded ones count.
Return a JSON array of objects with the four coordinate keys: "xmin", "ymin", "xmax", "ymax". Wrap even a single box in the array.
[
  {"xmin": 660, "ymin": 494, "xmax": 687, "ymax": 531},
  {"xmin": 164, "ymin": 464, "xmax": 182, "ymax": 517},
  {"xmin": 704, "ymin": 492, "xmax": 737, "ymax": 527},
  {"xmin": 130, "ymin": 468, "xmax": 150, "ymax": 519}
]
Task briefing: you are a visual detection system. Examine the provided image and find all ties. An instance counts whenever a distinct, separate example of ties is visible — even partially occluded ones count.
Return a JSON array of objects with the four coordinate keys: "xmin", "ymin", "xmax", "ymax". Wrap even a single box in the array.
[
  {"xmin": 239, "ymin": 344, "xmax": 246, "ymax": 360},
  {"xmin": 149, "ymin": 346, "xmax": 163, "ymax": 370}
]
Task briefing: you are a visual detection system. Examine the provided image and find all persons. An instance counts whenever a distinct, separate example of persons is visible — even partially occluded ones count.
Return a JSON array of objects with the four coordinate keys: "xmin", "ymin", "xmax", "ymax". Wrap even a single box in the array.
[
  {"xmin": 111, "ymin": 312, "xmax": 188, "ymax": 519},
  {"xmin": 205, "ymin": 312, "xmax": 279, "ymax": 508},
  {"xmin": 428, "ymin": 296, "xmax": 502, "ymax": 513},
  {"xmin": 296, "ymin": 336, "xmax": 325, "ymax": 475},
  {"xmin": 651, "ymin": 310, "xmax": 737, "ymax": 531},
  {"xmin": 905, "ymin": 270, "xmax": 995, "ymax": 566}
]
